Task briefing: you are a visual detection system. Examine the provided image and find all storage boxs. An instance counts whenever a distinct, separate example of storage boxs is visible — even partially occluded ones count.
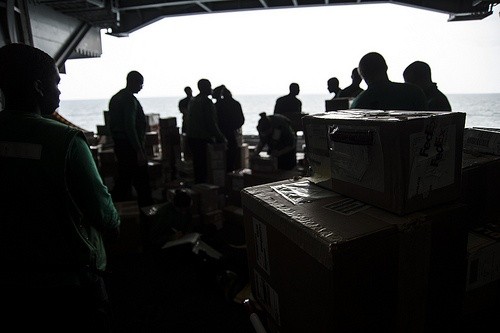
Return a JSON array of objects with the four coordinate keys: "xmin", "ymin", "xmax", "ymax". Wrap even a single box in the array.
[{"xmin": 83, "ymin": 98, "xmax": 500, "ymax": 333}]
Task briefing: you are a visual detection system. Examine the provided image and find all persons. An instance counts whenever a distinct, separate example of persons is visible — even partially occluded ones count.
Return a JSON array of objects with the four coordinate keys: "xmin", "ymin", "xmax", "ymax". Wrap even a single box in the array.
[
  {"xmin": 0, "ymin": 43, "xmax": 121, "ymax": 333},
  {"xmin": 350, "ymin": 53, "xmax": 427, "ymax": 111},
  {"xmin": 274, "ymin": 83, "xmax": 303, "ymax": 137},
  {"xmin": 179, "ymin": 78, "xmax": 245, "ymax": 183},
  {"xmin": 342, "ymin": 66, "xmax": 365, "ymax": 99},
  {"xmin": 108, "ymin": 71, "xmax": 147, "ymax": 201},
  {"xmin": 251, "ymin": 113, "xmax": 297, "ymax": 179},
  {"xmin": 401, "ymin": 61, "xmax": 452, "ymax": 110},
  {"xmin": 327, "ymin": 77, "xmax": 343, "ymax": 100}
]
